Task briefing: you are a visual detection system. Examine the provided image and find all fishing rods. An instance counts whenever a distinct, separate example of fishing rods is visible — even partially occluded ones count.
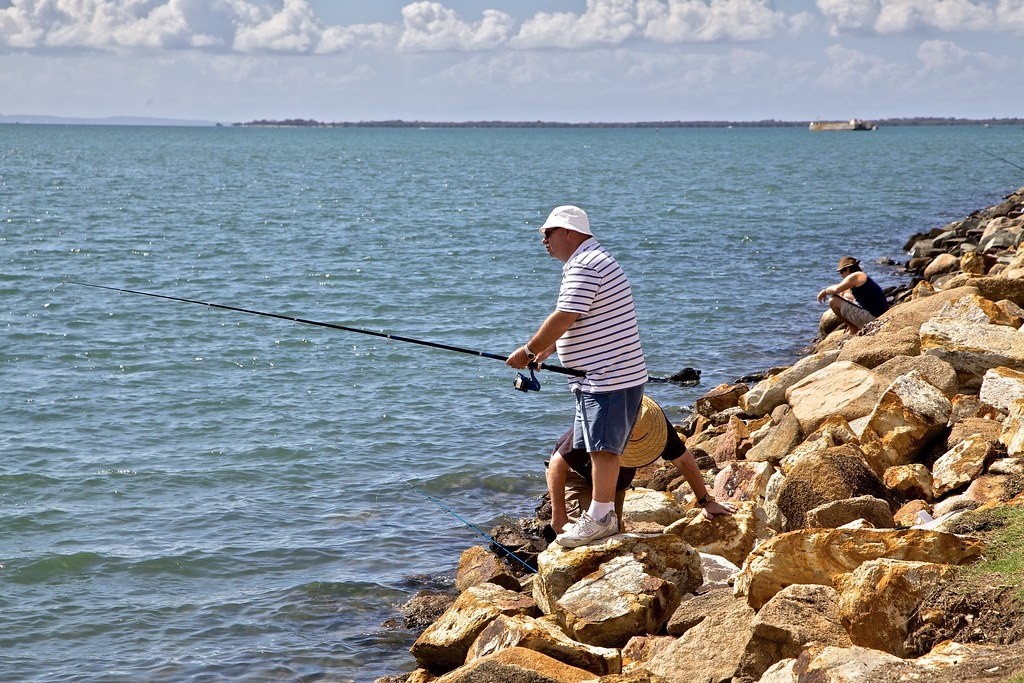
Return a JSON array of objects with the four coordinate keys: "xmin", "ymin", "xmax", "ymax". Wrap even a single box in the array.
[
  {"xmin": 66, "ymin": 279, "xmax": 591, "ymax": 395},
  {"xmin": 411, "ymin": 485, "xmax": 539, "ymax": 574},
  {"xmin": 982, "ymin": 149, "xmax": 1024, "ymax": 171}
]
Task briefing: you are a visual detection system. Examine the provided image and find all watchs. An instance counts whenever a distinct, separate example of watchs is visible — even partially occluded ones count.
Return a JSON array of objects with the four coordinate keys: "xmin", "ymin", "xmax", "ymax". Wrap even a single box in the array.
[
  {"xmin": 698, "ymin": 493, "xmax": 713, "ymax": 505},
  {"xmin": 524, "ymin": 344, "xmax": 536, "ymax": 361}
]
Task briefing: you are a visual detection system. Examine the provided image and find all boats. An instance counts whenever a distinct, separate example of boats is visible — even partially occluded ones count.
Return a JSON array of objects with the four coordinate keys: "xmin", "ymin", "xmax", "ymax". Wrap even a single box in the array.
[{"xmin": 808, "ymin": 121, "xmax": 879, "ymax": 131}]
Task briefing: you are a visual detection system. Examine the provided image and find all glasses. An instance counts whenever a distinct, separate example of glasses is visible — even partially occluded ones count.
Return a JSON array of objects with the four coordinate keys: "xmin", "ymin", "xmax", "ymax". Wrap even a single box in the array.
[
  {"xmin": 545, "ymin": 227, "xmax": 560, "ymax": 239},
  {"xmin": 839, "ymin": 268, "xmax": 845, "ymax": 274}
]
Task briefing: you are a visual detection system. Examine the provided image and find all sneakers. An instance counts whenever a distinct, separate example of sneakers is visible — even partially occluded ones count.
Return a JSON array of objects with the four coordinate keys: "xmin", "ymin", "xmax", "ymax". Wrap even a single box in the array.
[
  {"xmin": 555, "ymin": 510, "xmax": 616, "ymax": 547},
  {"xmin": 562, "ymin": 510, "xmax": 619, "ymax": 535}
]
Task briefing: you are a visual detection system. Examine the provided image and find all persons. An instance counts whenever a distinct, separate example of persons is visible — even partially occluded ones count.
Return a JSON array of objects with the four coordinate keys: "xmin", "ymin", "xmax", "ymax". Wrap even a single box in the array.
[
  {"xmin": 502, "ymin": 204, "xmax": 655, "ymax": 549},
  {"xmin": 542, "ymin": 394, "xmax": 738, "ymax": 537},
  {"xmin": 816, "ymin": 255, "xmax": 889, "ymax": 333}
]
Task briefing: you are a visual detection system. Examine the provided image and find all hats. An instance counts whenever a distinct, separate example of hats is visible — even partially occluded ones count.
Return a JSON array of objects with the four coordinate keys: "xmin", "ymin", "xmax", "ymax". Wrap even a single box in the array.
[
  {"xmin": 539, "ymin": 205, "xmax": 595, "ymax": 237},
  {"xmin": 618, "ymin": 394, "xmax": 667, "ymax": 469},
  {"xmin": 836, "ymin": 256, "xmax": 860, "ymax": 272}
]
